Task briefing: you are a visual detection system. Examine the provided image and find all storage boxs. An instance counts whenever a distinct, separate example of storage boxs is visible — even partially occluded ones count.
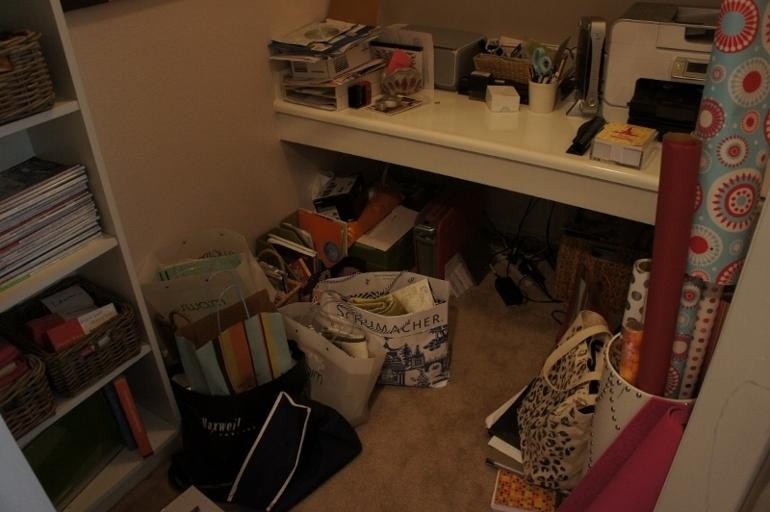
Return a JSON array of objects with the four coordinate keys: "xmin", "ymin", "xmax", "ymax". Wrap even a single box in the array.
[{"xmin": 0, "ymin": 276, "xmax": 141, "ymax": 441}]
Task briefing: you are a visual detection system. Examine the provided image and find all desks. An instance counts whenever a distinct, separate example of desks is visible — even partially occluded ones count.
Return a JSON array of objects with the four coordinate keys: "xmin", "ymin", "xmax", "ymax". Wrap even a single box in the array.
[{"xmin": 275, "ymin": 88, "xmax": 663, "ymax": 227}]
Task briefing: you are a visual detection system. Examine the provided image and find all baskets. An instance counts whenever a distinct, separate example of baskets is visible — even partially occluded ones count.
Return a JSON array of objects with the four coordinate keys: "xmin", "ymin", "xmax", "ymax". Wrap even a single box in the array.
[{"xmin": 0, "ymin": 278, "xmax": 141, "ymax": 441}]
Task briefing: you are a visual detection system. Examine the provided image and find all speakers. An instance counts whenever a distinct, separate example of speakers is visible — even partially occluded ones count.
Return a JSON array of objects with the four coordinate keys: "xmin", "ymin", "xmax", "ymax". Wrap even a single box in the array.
[{"xmin": 574, "ymin": 16, "xmax": 606, "ymax": 113}]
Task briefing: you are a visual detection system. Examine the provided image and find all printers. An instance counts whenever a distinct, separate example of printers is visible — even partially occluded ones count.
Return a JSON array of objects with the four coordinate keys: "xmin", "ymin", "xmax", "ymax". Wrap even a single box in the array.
[{"xmin": 602, "ymin": 0, "xmax": 723, "ymax": 143}]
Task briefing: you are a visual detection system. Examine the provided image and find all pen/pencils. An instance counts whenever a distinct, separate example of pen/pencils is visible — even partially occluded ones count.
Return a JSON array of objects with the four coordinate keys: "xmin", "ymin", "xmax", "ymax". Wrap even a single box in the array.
[
  {"xmin": 495, "ymin": 79, "xmax": 513, "ymax": 84},
  {"xmin": 528, "ymin": 48, "xmax": 569, "ymax": 83}
]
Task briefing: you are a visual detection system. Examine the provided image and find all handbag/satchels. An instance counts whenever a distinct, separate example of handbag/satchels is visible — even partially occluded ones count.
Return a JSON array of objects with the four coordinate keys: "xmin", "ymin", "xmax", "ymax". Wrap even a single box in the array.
[
  {"xmin": 518, "ymin": 310, "xmax": 611, "ymax": 492},
  {"xmin": 141, "ymin": 247, "xmax": 450, "ymax": 512}
]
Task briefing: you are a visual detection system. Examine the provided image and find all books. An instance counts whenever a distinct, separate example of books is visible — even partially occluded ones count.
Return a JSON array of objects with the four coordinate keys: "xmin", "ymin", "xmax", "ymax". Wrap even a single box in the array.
[
  {"xmin": 266, "ymin": 208, "xmax": 348, "ymax": 284},
  {"xmin": 101, "ymin": 372, "xmax": 155, "ymax": 459},
  {"xmin": 267, "ymin": 18, "xmax": 386, "ymax": 112},
  {"xmin": 0, "ymin": 157, "xmax": 118, "ymax": 359}
]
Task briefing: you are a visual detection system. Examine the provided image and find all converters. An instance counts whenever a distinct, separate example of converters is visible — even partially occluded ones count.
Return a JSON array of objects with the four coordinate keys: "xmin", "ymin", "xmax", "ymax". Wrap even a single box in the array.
[
  {"xmin": 495, "ymin": 276, "xmax": 523, "ymax": 306},
  {"xmin": 518, "ymin": 260, "xmax": 546, "ymax": 287}
]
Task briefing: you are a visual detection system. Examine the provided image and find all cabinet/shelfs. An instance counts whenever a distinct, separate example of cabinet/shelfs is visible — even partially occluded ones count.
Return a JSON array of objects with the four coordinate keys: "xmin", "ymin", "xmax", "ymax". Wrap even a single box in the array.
[{"xmin": 0, "ymin": 0, "xmax": 184, "ymax": 511}]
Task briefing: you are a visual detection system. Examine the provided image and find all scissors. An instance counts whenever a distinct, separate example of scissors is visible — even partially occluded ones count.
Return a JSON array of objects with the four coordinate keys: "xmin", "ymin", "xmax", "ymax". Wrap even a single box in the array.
[{"xmin": 533, "ymin": 47, "xmax": 551, "ymax": 83}]
